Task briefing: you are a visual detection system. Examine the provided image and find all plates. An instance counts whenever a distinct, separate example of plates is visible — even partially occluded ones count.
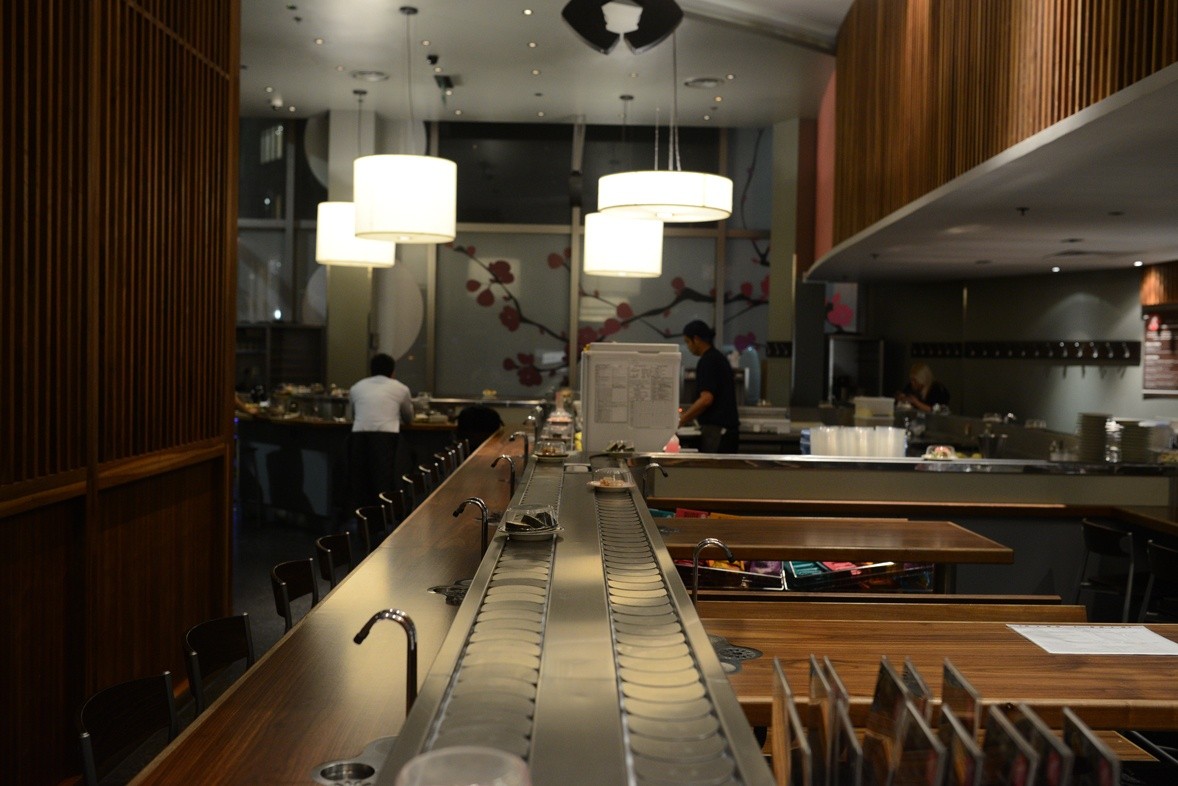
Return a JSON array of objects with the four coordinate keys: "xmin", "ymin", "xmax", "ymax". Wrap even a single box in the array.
[
  {"xmin": 541, "ymin": 435, "xmax": 568, "ymax": 441},
  {"xmin": 547, "ymin": 418, "xmax": 572, "ymax": 423},
  {"xmin": 496, "ymin": 525, "xmax": 565, "ymax": 541},
  {"xmin": 921, "ymin": 455, "xmax": 959, "ymax": 461},
  {"xmin": 586, "ymin": 480, "xmax": 635, "ymax": 492},
  {"xmin": 1074, "ymin": 407, "xmax": 1172, "ymax": 463},
  {"xmin": 532, "ymin": 453, "xmax": 569, "ymax": 462}
]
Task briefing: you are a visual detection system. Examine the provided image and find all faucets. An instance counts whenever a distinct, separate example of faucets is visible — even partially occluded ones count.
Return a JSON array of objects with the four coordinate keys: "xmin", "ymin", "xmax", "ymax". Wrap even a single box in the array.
[
  {"xmin": 352, "ymin": 608, "xmax": 418, "ymax": 720},
  {"xmin": 641, "ymin": 462, "xmax": 670, "ymax": 504},
  {"xmin": 452, "ymin": 497, "xmax": 489, "ymax": 563},
  {"xmin": 691, "ymin": 537, "xmax": 737, "ymax": 603},
  {"xmin": 522, "ymin": 415, "xmax": 539, "ymax": 450},
  {"xmin": 490, "ymin": 453, "xmax": 516, "ymax": 501},
  {"xmin": 531, "ymin": 399, "xmax": 548, "ymax": 427},
  {"xmin": 1003, "ymin": 413, "xmax": 1018, "ymax": 425},
  {"xmin": 508, "ymin": 430, "xmax": 529, "ymax": 471}
]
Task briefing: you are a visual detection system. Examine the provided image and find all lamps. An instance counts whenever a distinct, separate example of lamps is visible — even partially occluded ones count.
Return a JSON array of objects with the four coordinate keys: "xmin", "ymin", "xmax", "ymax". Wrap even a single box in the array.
[
  {"xmin": 597, "ymin": 23, "xmax": 732, "ymax": 222},
  {"xmin": 583, "ymin": 95, "xmax": 665, "ymax": 279},
  {"xmin": 353, "ymin": 5, "xmax": 458, "ymax": 246},
  {"xmin": 314, "ymin": 90, "xmax": 396, "ymax": 268}
]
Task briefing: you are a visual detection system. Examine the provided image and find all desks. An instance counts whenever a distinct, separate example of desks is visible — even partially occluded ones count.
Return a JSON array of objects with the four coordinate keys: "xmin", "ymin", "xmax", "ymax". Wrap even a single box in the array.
[
  {"xmin": 233, "ymin": 411, "xmax": 459, "ymax": 531},
  {"xmin": 648, "ymin": 503, "xmax": 1178, "ymax": 732}
]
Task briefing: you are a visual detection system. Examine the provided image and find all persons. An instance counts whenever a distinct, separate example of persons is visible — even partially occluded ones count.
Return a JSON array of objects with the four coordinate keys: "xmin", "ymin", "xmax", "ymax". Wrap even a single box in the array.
[
  {"xmin": 679, "ymin": 319, "xmax": 739, "ymax": 454},
  {"xmin": 345, "ymin": 354, "xmax": 414, "ymax": 533},
  {"xmin": 895, "ymin": 363, "xmax": 950, "ymax": 413},
  {"xmin": 235, "ymin": 365, "xmax": 261, "ymax": 418}
]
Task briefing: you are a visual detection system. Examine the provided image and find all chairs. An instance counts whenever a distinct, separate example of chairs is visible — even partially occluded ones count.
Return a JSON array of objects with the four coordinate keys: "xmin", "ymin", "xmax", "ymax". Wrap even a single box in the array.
[
  {"xmin": 1135, "ymin": 537, "xmax": 1177, "ymax": 624},
  {"xmin": 76, "ymin": 437, "xmax": 470, "ymax": 786},
  {"xmin": 1073, "ymin": 518, "xmax": 1147, "ymax": 624}
]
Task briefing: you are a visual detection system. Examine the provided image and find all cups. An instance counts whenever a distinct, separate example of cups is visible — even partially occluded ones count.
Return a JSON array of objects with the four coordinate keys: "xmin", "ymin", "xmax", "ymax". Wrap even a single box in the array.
[{"xmin": 417, "ymin": 393, "xmax": 432, "ymax": 408}]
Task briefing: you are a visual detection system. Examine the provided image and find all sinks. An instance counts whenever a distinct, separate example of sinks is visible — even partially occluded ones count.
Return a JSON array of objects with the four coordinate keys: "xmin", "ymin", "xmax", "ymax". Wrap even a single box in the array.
[
  {"xmin": 715, "ymin": 645, "xmax": 763, "ymax": 663},
  {"xmin": 427, "ymin": 585, "xmax": 466, "ymax": 596},
  {"xmin": 311, "ymin": 759, "xmax": 384, "ymax": 786}
]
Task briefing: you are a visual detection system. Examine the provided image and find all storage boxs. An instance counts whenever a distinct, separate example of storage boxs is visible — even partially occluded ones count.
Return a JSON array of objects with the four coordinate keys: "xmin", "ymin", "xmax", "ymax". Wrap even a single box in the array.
[{"xmin": 784, "ymin": 557, "xmax": 936, "ymax": 592}]
